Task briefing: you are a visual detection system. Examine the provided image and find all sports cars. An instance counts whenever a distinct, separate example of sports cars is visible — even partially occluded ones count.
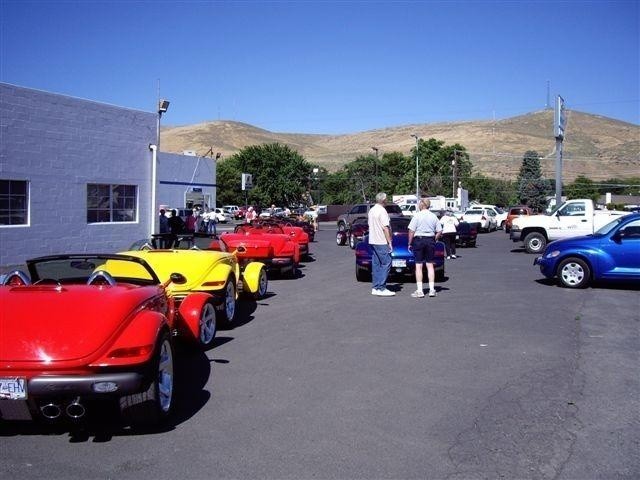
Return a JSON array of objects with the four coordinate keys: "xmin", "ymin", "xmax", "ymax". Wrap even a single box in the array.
[
  {"xmin": 355, "ymin": 217, "xmax": 445, "ymax": 282},
  {"xmin": 209, "ymin": 222, "xmax": 300, "ymax": 278},
  {"xmin": 91, "ymin": 233, "xmax": 268, "ymax": 328},
  {"xmin": 0, "ymin": 253, "xmax": 218, "ymax": 431}
]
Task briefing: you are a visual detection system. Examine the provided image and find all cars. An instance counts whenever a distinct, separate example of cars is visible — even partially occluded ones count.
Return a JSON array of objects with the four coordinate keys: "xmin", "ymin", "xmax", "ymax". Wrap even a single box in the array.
[{"xmin": 533, "ymin": 213, "xmax": 640, "ymax": 288}]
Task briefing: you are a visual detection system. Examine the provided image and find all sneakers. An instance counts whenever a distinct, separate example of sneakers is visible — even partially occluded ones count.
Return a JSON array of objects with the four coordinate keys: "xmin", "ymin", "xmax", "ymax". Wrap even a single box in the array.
[
  {"xmin": 446, "ymin": 254, "xmax": 457, "ymax": 259},
  {"xmin": 375, "ymin": 289, "xmax": 396, "ymax": 297},
  {"xmin": 371, "ymin": 289, "xmax": 377, "ymax": 295},
  {"xmin": 428, "ymin": 289, "xmax": 437, "ymax": 297},
  {"xmin": 409, "ymin": 291, "xmax": 426, "ymax": 298}
]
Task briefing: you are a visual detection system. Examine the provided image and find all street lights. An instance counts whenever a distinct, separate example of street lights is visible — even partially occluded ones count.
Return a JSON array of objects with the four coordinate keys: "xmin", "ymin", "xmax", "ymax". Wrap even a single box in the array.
[
  {"xmin": 410, "ymin": 133, "xmax": 419, "ymax": 213},
  {"xmin": 371, "ymin": 146, "xmax": 379, "ymax": 195}
]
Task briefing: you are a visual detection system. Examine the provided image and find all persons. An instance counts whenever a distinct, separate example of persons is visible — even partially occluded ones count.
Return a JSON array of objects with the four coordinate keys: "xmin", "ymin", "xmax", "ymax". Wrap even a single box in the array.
[
  {"xmin": 368, "ymin": 192, "xmax": 396, "ymax": 296},
  {"xmin": 270, "ymin": 205, "xmax": 318, "ymax": 231},
  {"xmin": 231, "ymin": 206, "xmax": 260, "ymax": 222},
  {"xmin": 408, "ymin": 199, "xmax": 443, "ymax": 298},
  {"xmin": 159, "ymin": 207, "xmax": 217, "ymax": 235},
  {"xmin": 439, "ymin": 211, "xmax": 460, "ymax": 260}
]
